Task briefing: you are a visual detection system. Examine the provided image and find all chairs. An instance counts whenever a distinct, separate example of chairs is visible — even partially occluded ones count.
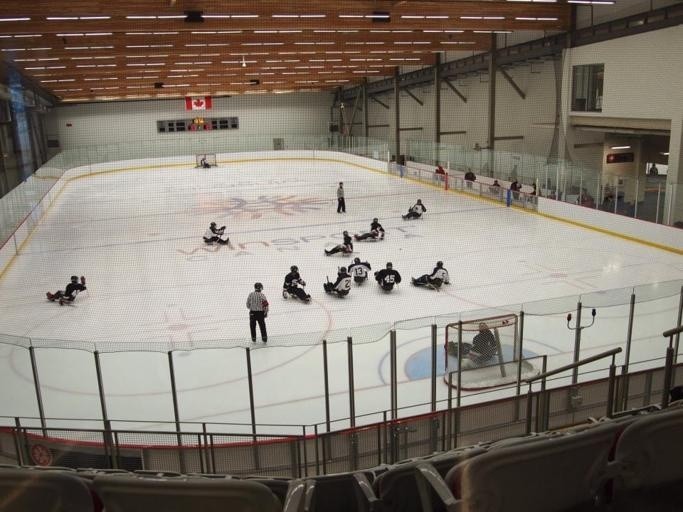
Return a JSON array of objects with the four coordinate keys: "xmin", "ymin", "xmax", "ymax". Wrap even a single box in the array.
[{"xmin": 0, "ymin": 398, "xmax": 683, "ymax": 511}]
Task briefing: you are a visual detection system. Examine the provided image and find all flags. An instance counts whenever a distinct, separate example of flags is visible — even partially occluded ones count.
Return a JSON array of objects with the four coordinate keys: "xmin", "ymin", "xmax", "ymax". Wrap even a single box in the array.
[{"xmin": 183, "ymin": 95, "xmax": 212, "ymax": 111}]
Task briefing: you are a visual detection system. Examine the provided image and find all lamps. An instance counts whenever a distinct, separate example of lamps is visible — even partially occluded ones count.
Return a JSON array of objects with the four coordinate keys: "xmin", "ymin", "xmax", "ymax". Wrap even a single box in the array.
[
  {"xmin": 340, "ymin": 102, "xmax": 345, "ymax": 109},
  {"xmin": 242, "ymin": 55, "xmax": 246, "ymax": 68}
]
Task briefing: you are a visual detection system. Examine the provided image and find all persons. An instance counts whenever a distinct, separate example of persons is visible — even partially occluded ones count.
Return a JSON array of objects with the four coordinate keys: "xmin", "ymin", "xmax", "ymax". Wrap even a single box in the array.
[
  {"xmin": 202, "ymin": 221, "xmax": 230, "ymax": 247},
  {"xmin": 510, "ymin": 180, "xmax": 522, "ymax": 200},
  {"xmin": 347, "ymin": 257, "xmax": 371, "ymax": 286},
  {"xmin": 648, "ymin": 162, "xmax": 658, "ymax": 176},
  {"xmin": 45, "ymin": 274, "xmax": 85, "ymax": 305},
  {"xmin": 434, "ymin": 165, "xmax": 445, "ymax": 181},
  {"xmin": 373, "ymin": 261, "xmax": 401, "ymax": 291},
  {"xmin": 335, "ymin": 182, "xmax": 345, "ymax": 214},
  {"xmin": 323, "ymin": 266, "xmax": 351, "ymax": 297},
  {"xmin": 489, "ymin": 179, "xmax": 500, "ymax": 195},
  {"xmin": 467, "ymin": 321, "xmax": 498, "ymax": 363},
  {"xmin": 323, "ymin": 230, "xmax": 353, "ymax": 256},
  {"xmin": 576, "ymin": 188, "xmax": 593, "ymax": 207},
  {"xmin": 200, "ymin": 158, "xmax": 211, "ymax": 168},
  {"xmin": 463, "ymin": 168, "xmax": 476, "ymax": 188},
  {"xmin": 353, "ymin": 217, "xmax": 384, "ymax": 241},
  {"xmin": 401, "ymin": 199, "xmax": 426, "ymax": 220},
  {"xmin": 547, "ymin": 190, "xmax": 556, "ymax": 199},
  {"xmin": 527, "ymin": 183, "xmax": 541, "ymax": 203},
  {"xmin": 410, "ymin": 260, "xmax": 449, "ymax": 289},
  {"xmin": 246, "ymin": 282, "xmax": 268, "ymax": 342},
  {"xmin": 282, "ymin": 265, "xmax": 310, "ymax": 303}
]
